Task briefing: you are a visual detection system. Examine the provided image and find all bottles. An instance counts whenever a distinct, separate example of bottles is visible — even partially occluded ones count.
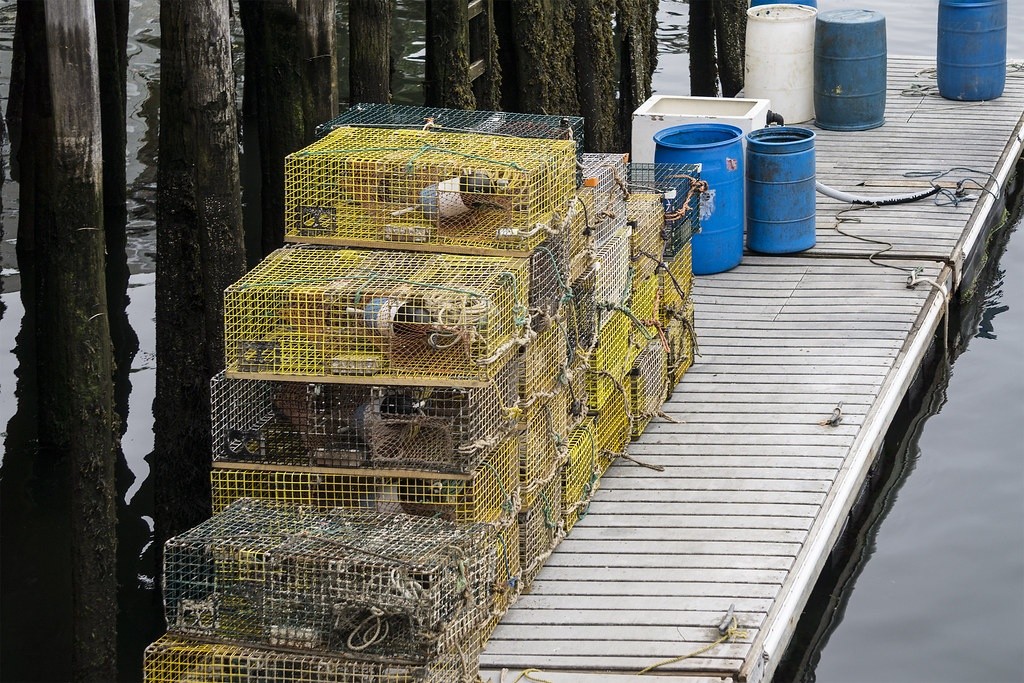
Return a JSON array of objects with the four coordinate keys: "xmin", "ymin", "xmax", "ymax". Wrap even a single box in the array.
[
  {"xmin": 415, "ymin": 174, "xmax": 509, "ymax": 226},
  {"xmin": 364, "ymin": 480, "xmax": 434, "ymax": 511},
  {"xmin": 362, "ymin": 298, "xmax": 440, "ymax": 337},
  {"xmin": 351, "ymin": 396, "xmax": 425, "ymax": 435}
]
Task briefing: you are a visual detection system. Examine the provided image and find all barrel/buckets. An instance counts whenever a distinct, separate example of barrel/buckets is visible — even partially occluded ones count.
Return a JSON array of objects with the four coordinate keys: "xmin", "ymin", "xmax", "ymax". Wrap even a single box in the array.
[
  {"xmin": 814, "ymin": 8, "xmax": 887, "ymax": 131},
  {"xmin": 653, "ymin": 124, "xmax": 744, "ymax": 275},
  {"xmin": 744, "ymin": 4, "xmax": 819, "ymax": 124},
  {"xmin": 745, "ymin": 126, "xmax": 815, "ymax": 255},
  {"xmin": 750, "ymin": 0, "xmax": 816, "ymax": 9},
  {"xmin": 937, "ymin": 0, "xmax": 1007, "ymax": 102}
]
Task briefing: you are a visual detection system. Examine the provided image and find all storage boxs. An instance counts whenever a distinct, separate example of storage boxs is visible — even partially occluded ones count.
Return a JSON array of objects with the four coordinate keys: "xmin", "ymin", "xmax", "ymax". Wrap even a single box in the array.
[{"xmin": 631, "ymin": 93, "xmax": 770, "ymax": 233}]
